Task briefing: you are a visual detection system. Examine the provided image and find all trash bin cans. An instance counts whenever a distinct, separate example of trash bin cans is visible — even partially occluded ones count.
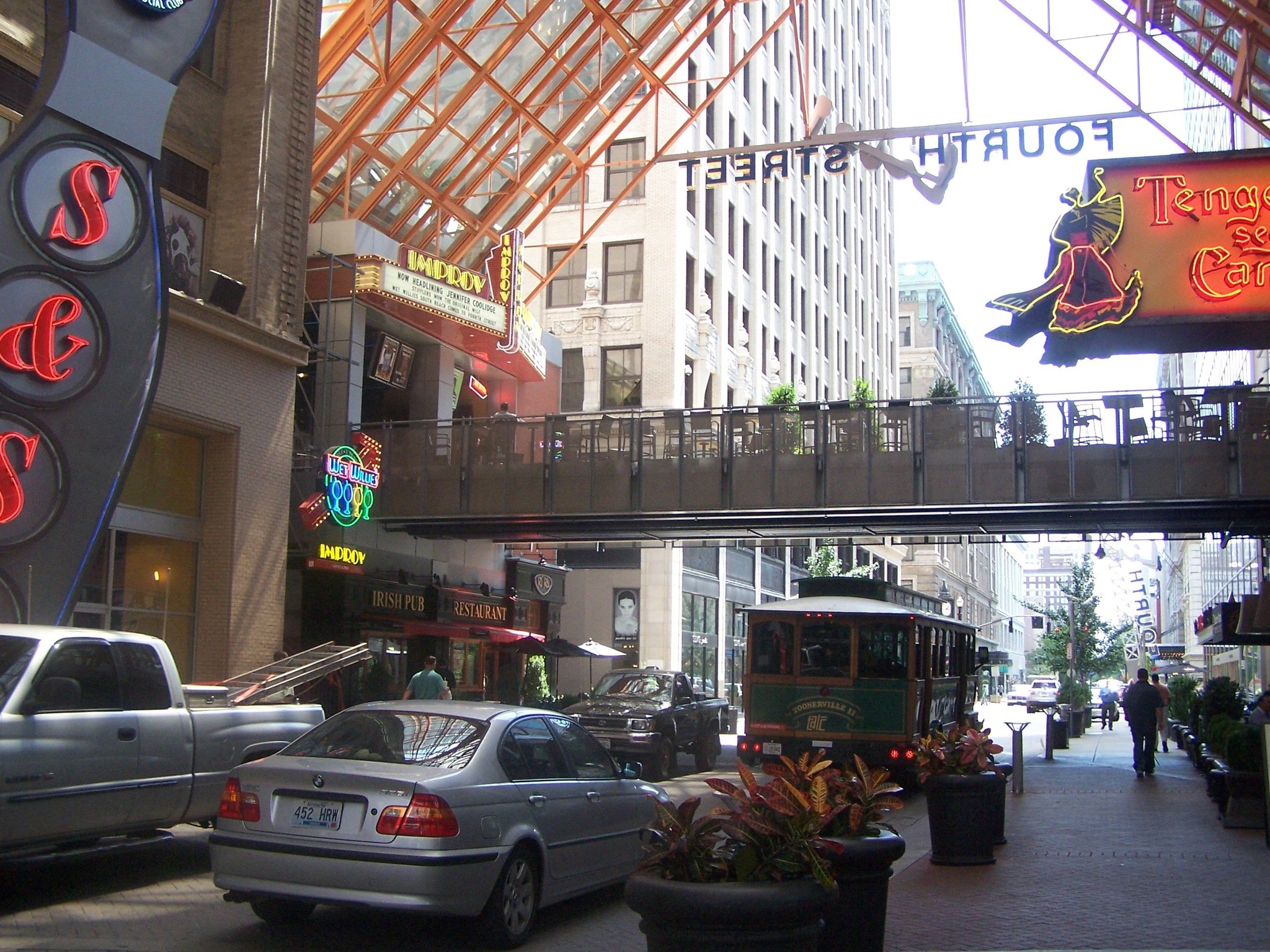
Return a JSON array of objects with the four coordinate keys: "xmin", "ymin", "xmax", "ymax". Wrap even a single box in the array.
[
  {"xmin": 1053, "ymin": 719, "xmax": 1069, "ymax": 749},
  {"xmin": 720, "ymin": 705, "xmax": 738, "ymax": 734}
]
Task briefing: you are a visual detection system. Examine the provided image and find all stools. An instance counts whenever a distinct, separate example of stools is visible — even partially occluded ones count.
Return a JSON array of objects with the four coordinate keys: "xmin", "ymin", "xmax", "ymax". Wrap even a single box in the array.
[{"xmin": 662, "ymin": 399, "xmax": 910, "ymax": 460}]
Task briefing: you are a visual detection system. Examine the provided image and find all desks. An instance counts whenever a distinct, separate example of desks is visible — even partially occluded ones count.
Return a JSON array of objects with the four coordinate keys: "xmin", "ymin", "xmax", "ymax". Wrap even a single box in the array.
[
  {"xmin": 1102, "ymin": 394, "xmax": 1144, "ymax": 445},
  {"xmin": 1201, "ymin": 385, "xmax": 1253, "ymax": 436},
  {"xmin": 745, "ymin": 413, "xmax": 797, "ymax": 445},
  {"xmin": 519, "ymin": 422, "xmax": 584, "ymax": 463}
]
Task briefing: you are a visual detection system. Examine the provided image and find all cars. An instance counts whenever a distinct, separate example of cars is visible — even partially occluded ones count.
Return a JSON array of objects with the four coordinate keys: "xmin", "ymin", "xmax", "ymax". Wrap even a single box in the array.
[
  {"xmin": 1088, "ymin": 686, "xmax": 1120, "ymax": 722},
  {"xmin": 1006, "ymin": 684, "xmax": 1031, "ymax": 706},
  {"xmin": 207, "ymin": 699, "xmax": 680, "ymax": 950},
  {"xmin": 1117, "ymin": 684, "xmax": 1130, "ymax": 707}
]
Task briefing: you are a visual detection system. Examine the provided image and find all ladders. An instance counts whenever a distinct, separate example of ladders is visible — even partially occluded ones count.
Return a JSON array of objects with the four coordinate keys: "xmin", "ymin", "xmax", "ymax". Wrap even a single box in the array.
[{"xmin": 214, "ymin": 641, "xmax": 373, "ymax": 706}]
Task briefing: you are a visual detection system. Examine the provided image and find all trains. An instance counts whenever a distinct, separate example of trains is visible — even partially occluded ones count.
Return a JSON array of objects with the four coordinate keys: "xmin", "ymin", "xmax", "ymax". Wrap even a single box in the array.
[{"xmin": 733, "ymin": 576, "xmax": 990, "ymax": 799}]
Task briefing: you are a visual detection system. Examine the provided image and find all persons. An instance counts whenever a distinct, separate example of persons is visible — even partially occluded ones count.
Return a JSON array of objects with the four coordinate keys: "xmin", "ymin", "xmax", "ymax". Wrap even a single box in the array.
[
  {"xmin": 1099, "ymin": 668, "xmax": 1172, "ymax": 778},
  {"xmin": 376, "ymin": 347, "xmax": 410, "ymax": 386},
  {"xmin": 402, "ymin": 655, "xmax": 457, "ymax": 700},
  {"xmin": 267, "ymin": 651, "xmax": 294, "ymax": 703},
  {"xmin": 975, "ymin": 683, "xmax": 1005, "ymax": 705},
  {"xmin": 1248, "ymin": 694, "xmax": 1270, "ymax": 725},
  {"xmin": 614, "ymin": 591, "xmax": 638, "ymax": 636},
  {"xmin": 482, "ymin": 402, "xmax": 525, "ymax": 442}
]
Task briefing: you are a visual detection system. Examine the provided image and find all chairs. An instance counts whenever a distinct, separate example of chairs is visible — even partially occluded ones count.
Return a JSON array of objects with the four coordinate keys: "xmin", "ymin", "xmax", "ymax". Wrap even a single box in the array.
[
  {"xmin": 972, "ymin": 397, "xmax": 1001, "ymax": 449},
  {"xmin": 578, "ymin": 414, "xmax": 657, "ymax": 459},
  {"xmin": 1058, "ymin": 394, "xmax": 1213, "ymax": 446},
  {"xmin": 492, "ymin": 421, "xmax": 517, "ymax": 453}
]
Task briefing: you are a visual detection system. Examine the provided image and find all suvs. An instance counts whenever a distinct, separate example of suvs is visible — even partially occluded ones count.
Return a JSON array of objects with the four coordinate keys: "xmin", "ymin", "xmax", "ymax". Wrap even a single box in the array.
[{"xmin": 1025, "ymin": 679, "xmax": 1062, "ymax": 713}]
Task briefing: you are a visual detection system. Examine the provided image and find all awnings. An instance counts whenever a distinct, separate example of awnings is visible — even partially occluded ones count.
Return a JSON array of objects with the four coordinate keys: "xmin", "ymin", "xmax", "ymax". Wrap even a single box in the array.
[{"xmin": 358, "ymin": 611, "xmax": 545, "ymax": 645}]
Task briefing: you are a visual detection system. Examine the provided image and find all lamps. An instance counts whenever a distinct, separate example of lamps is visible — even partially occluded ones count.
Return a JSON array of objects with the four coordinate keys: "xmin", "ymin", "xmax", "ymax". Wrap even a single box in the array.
[
  {"xmin": 1094, "ymin": 532, "xmax": 1106, "ymax": 559},
  {"xmin": 1252, "ymin": 581, "xmax": 1270, "ymax": 629},
  {"xmin": 411, "ymin": 573, "xmax": 442, "ymax": 591},
  {"xmin": 1236, "ymin": 595, "xmax": 1270, "ymax": 634},
  {"xmin": 491, "ymin": 587, "xmax": 519, "ymax": 603},
  {"xmin": 520, "ymin": 552, "xmax": 547, "ymax": 565},
  {"xmin": 381, "ymin": 569, "xmax": 408, "ymax": 586},
  {"xmin": 462, "ymin": 582, "xmax": 489, "ymax": 597},
  {"xmin": 546, "ymin": 559, "xmax": 567, "ymax": 568}
]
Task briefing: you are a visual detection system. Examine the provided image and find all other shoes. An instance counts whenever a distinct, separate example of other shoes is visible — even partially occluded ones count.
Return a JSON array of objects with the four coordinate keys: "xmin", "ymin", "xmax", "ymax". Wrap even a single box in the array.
[
  {"xmin": 1145, "ymin": 769, "xmax": 1154, "ymax": 775},
  {"xmin": 1109, "ymin": 728, "xmax": 1113, "ymax": 731},
  {"xmin": 1137, "ymin": 770, "xmax": 1144, "ymax": 777},
  {"xmin": 1154, "ymin": 748, "xmax": 1157, "ymax": 751},
  {"xmin": 1101, "ymin": 723, "xmax": 1107, "ymax": 729},
  {"xmin": 1162, "ymin": 741, "xmax": 1168, "ymax": 752}
]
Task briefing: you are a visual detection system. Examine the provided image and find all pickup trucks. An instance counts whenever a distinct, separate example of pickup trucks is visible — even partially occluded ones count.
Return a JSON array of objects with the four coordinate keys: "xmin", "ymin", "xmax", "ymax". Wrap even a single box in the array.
[
  {"xmin": 0, "ymin": 622, "xmax": 327, "ymax": 876},
  {"xmin": 558, "ymin": 668, "xmax": 730, "ymax": 782}
]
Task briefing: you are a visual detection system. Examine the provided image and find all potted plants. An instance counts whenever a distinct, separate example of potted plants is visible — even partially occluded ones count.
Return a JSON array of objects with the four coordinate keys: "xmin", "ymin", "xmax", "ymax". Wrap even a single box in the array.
[
  {"xmin": 1165, "ymin": 674, "xmax": 1266, "ymax": 829},
  {"xmin": 910, "ymin": 722, "xmax": 1006, "ymax": 868},
  {"xmin": 625, "ymin": 748, "xmax": 906, "ymax": 952}
]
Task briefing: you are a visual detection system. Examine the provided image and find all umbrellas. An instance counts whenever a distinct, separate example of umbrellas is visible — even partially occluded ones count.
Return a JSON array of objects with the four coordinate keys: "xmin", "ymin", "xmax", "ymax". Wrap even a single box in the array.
[
  {"xmin": 497, "ymin": 633, "xmax": 626, "ymax": 699},
  {"xmin": 1151, "ymin": 662, "xmax": 1209, "ymax": 682}
]
